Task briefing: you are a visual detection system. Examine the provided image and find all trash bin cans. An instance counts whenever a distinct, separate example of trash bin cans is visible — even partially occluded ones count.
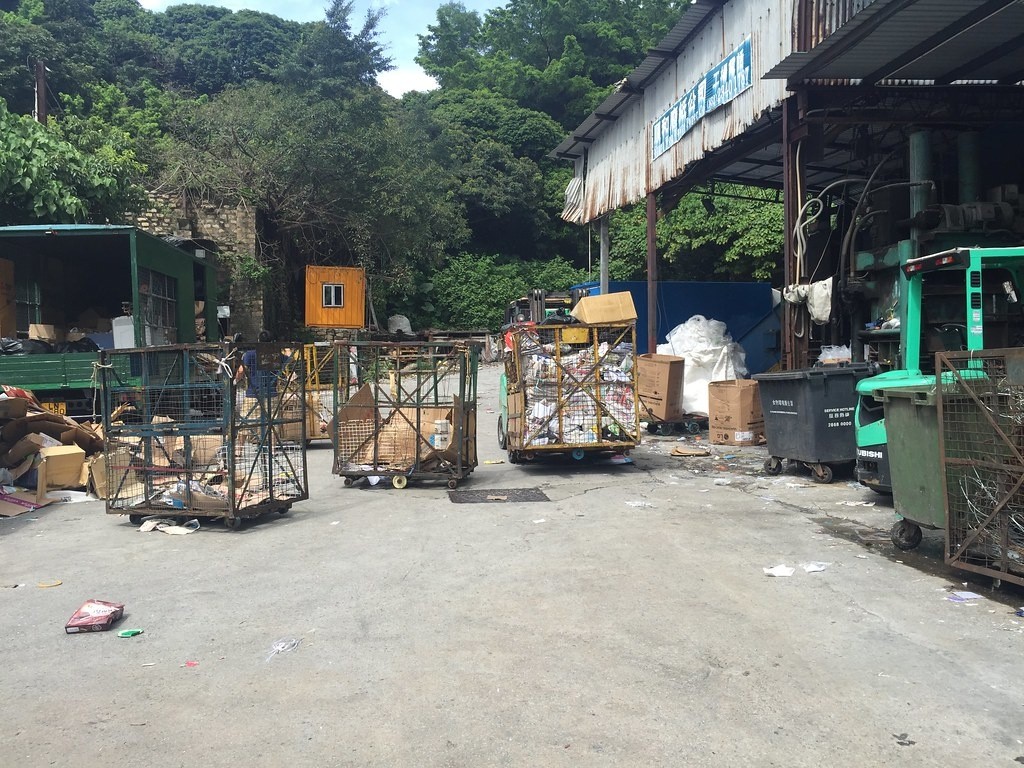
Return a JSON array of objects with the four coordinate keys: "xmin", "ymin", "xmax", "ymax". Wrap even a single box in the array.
[
  {"xmin": 751, "ymin": 360, "xmax": 881, "ymax": 484},
  {"xmin": 872, "ymin": 371, "xmax": 995, "ymax": 550}
]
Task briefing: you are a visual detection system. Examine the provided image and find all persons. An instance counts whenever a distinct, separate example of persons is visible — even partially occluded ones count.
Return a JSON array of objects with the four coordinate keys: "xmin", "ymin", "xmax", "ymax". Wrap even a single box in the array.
[
  {"xmin": 232, "ymin": 330, "xmax": 294, "ymax": 457},
  {"xmin": 133, "ymin": 283, "xmax": 154, "ymax": 317}
]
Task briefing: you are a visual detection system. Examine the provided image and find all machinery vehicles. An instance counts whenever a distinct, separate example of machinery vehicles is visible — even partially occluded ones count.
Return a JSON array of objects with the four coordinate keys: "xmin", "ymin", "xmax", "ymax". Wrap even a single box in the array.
[{"xmin": 851, "ymin": 247, "xmax": 1024, "ymax": 493}]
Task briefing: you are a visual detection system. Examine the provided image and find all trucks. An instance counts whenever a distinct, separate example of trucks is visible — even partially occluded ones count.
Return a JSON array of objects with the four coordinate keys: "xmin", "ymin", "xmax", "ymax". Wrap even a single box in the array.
[{"xmin": 0, "ymin": 222, "xmax": 228, "ymax": 421}]
